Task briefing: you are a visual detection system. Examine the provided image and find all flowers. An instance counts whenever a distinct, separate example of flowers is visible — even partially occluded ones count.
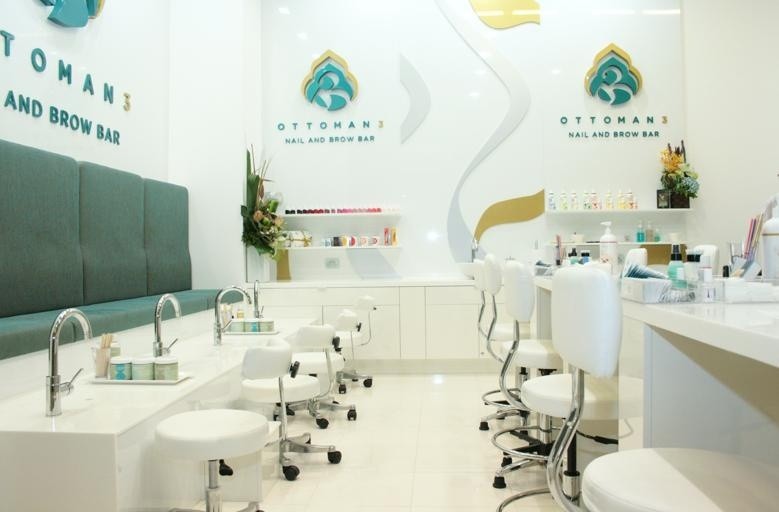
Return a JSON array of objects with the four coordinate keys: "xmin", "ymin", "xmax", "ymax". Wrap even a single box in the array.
[
  {"xmin": 238, "ymin": 142, "xmax": 297, "ymax": 261},
  {"xmin": 656, "ymin": 138, "xmax": 702, "ymax": 200}
]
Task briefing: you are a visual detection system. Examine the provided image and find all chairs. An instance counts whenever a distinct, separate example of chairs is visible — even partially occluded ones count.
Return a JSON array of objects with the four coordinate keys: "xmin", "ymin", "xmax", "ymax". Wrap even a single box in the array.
[
  {"xmin": 546, "ymin": 266, "xmax": 776, "ymax": 512},
  {"xmin": 156, "ymin": 340, "xmax": 291, "ymax": 512},
  {"xmin": 242, "ymin": 324, "xmax": 341, "ymax": 481},
  {"xmin": 274, "ymin": 294, "xmax": 377, "ymax": 428},
  {"xmin": 462, "ymin": 253, "xmax": 619, "ymax": 512}
]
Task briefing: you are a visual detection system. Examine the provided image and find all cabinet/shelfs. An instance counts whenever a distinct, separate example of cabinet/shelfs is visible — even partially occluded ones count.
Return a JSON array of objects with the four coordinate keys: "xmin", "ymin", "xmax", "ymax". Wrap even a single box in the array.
[
  {"xmin": 270, "ymin": 202, "xmax": 404, "ymax": 253},
  {"xmin": 542, "ymin": 205, "xmax": 700, "ymax": 252}
]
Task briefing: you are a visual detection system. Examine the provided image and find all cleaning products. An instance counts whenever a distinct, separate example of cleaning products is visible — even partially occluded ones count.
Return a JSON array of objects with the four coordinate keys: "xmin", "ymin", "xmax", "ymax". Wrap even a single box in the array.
[{"xmin": 636, "ymin": 219, "xmax": 661, "ymax": 242}]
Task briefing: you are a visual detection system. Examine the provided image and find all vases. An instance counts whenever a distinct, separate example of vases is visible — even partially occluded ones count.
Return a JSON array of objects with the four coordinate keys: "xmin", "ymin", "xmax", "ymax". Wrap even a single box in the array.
[
  {"xmin": 244, "ymin": 242, "xmax": 272, "ymax": 285},
  {"xmin": 654, "ymin": 185, "xmax": 691, "ymax": 211}
]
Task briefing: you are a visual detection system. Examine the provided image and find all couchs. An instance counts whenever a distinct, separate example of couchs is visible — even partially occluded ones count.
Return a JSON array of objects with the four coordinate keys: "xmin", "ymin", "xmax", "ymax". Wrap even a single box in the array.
[{"xmin": 0, "ymin": 139, "xmax": 244, "ymax": 364}]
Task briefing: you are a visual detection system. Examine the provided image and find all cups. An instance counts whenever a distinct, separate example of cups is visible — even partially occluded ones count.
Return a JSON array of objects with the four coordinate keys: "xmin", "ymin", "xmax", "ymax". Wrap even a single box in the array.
[
  {"xmin": 90, "ymin": 346, "xmax": 110, "ymax": 378},
  {"xmin": 320, "ymin": 234, "xmax": 381, "ymax": 247},
  {"xmin": 220, "ymin": 305, "xmax": 231, "ymax": 330}
]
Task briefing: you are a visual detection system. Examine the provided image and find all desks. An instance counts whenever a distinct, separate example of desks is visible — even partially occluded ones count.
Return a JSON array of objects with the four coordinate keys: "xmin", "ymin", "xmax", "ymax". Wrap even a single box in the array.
[{"xmin": 529, "ymin": 275, "xmax": 777, "ymax": 468}]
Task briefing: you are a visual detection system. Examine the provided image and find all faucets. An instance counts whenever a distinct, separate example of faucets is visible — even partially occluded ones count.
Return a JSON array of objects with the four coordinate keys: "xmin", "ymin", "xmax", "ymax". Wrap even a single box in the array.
[
  {"xmin": 44, "ymin": 308, "xmax": 94, "ymax": 418},
  {"xmin": 254, "ymin": 281, "xmax": 265, "ymax": 317},
  {"xmin": 214, "ymin": 284, "xmax": 252, "ymax": 345},
  {"xmin": 153, "ymin": 293, "xmax": 181, "ymax": 359}
]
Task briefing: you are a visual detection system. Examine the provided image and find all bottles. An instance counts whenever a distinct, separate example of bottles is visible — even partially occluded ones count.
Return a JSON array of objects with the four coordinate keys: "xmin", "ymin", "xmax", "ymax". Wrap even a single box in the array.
[
  {"xmin": 571, "ymin": 233, "xmax": 579, "ymax": 242},
  {"xmin": 685, "ymin": 254, "xmax": 703, "ymax": 282},
  {"xmin": 547, "ymin": 188, "xmax": 633, "ymax": 209},
  {"xmin": 762, "ymin": 207, "xmax": 778, "ymax": 286},
  {"xmin": 579, "ymin": 249, "xmax": 592, "ymax": 263},
  {"xmin": 237, "ymin": 308, "xmax": 244, "ymax": 318}
]
